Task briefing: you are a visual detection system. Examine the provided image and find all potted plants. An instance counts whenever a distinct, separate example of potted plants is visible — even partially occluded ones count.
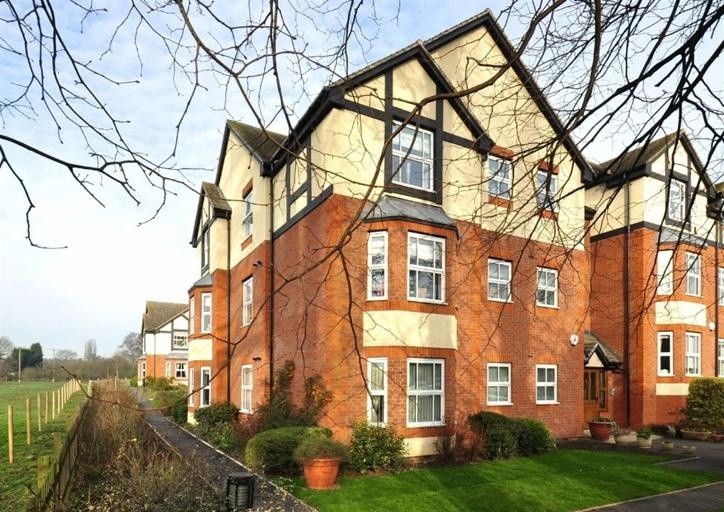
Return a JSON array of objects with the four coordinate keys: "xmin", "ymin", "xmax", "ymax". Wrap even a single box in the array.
[
  {"xmin": 585, "ymin": 416, "xmax": 615, "ymax": 441},
  {"xmin": 679, "ymin": 418, "xmax": 712, "ymax": 440},
  {"xmin": 291, "ymin": 436, "xmax": 349, "ymax": 490},
  {"xmin": 635, "ymin": 428, "xmax": 653, "ymax": 449},
  {"xmin": 661, "ymin": 438, "xmax": 673, "ymax": 450},
  {"xmin": 612, "ymin": 421, "xmax": 636, "ymax": 444}
]
